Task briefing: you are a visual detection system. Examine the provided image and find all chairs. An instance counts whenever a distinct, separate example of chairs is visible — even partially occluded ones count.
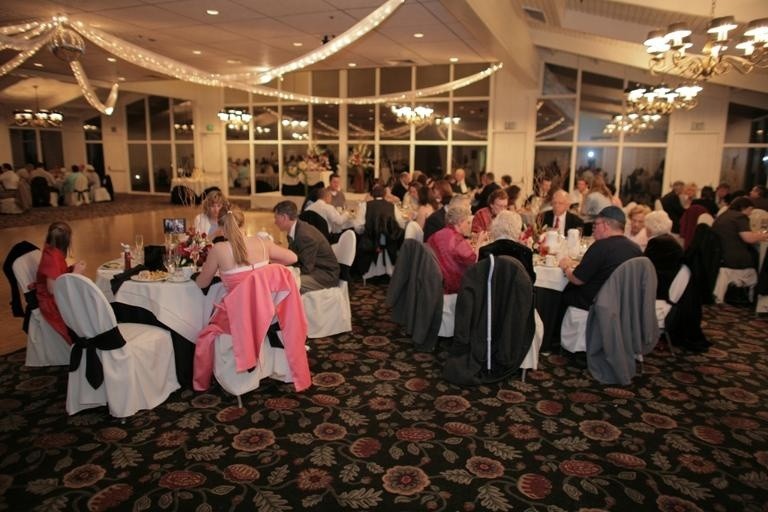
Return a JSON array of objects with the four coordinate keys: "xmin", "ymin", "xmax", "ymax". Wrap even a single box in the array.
[
  {"xmin": 1, "ymin": 178, "xmax": 27, "ymax": 215},
  {"xmin": 63, "ymin": 175, "xmax": 90, "ymax": 206},
  {"xmin": 697, "ymin": 213, "xmax": 758, "ymax": 305},
  {"xmin": 558, "ymin": 256, "xmax": 658, "ymax": 375},
  {"xmin": 12, "ymin": 108, "xmax": 64, "ymax": 129},
  {"xmin": 302, "ymin": 230, "xmax": 357, "ymax": 338},
  {"xmin": 654, "ymin": 264, "xmax": 692, "ymax": 355},
  {"xmin": 452, "ymin": 255, "xmax": 544, "ymax": 382},
  {"xmin": 359, "ymin": 212, "xmax": 402, "ymax": 287},
  {"xmin": 12, "ymin": 249, "xmax": 75, "ymax": 368},
  {"xmin": 52, "ymin": 272, "xmax": 182, "ymax": 418},
  {"xmin": 210, "ymin": 262, "xmax": 302, "ymax": 410},
  {"xmin": 404, "ymin": 220, "xmax": 424, "ymax": 244},
  {"xmin": 401, "ymin": 237, "xmax": 458, "ymax": 351},
  {"xmin": 30, "ymin": 176, "xmax": 58, "ymax": 207},
  {"xmin": 94, "ymin": 174, "xmax": 113, "ymax": 202}
]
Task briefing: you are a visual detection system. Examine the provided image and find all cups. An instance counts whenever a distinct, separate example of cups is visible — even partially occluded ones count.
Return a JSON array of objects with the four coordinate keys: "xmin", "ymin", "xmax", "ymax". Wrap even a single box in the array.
[
  {"xmin": 163, "ymin": 254, "xmax": 175, "ymax": 276},
  {"xmin": 486, "ymin": 225, "xmax": 493, "ymax": 244},
  {"xmin": 546, "ymin": 255, "xmax": 554, "ymax": 267},
  {"xmin": 136, "ymin": 234, "xmax": 143, "ymax": 257}
]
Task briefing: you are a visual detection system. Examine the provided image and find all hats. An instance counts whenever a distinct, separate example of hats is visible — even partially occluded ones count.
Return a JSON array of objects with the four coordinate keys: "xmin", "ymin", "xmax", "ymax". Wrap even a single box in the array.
[{"xmin": 589, "ymin": 206, "xmax": 626, "ymax": 225}]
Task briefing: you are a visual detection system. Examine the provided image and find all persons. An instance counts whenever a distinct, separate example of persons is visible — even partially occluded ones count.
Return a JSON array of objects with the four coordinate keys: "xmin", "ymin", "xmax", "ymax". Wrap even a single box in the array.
[
  {"xmin": 196, "ymin": 204, "xmax": 299, "ymax": 334},
  {"xmin": 660, "ymin": 180, "xmax": 686, "ymax": 232},
  {"xmin": 524, "ymin": 176, "xmax": 553, "ymax": 208},
  {"xmin": 422, "ymin": 194, "xmax": 471, "ymax": 242},
  {"xmin": 354, "ymin": 183, "xmax": 406, "ymax": 236},
  {"xmin": 304, "ymin": 186, "xmax": 351, "ymax": 245},
  {"xmin": 409, "ymin": 181, "xmax": 424, "ymax": 222},
  {"xmin": 568, "ymin": 177, "xmax": 589, "ymax": 210},
  {"xmin": 16, "ymin": 162, "xmax": 57, "ymax": 192},
  {"xmin": 432, "ymin": 180, "xmax": 454, "ymax": 205},
  {"xmin": 59, "ymin": 164, "xmax": 101, "ymax": 196},
  {"xmin": 500, "ymin": 175, "xmax": 512, "ymax": 189},
  {"xmin": 711, "ymin": 196, "xmax": 768, "ymax": 269},
  {"xmin": 749, "ymin": 185, "xmax": 768, "ymax": 211},
  {"xmin": 558, "ymin": 206, "xmax": 644, "ymax": 311},
  {"xmin": 425, "ymin": 166, "xmax": 456, "ymax": 210},
  {"xmin": 0, "ymin": 162, "xmax": 20, "ymax": 199},
  {"xmin": 580, "ymin": 176, "xmax": 614, "ymax": 216},
  {"xmin": 391, "ymin": 172, "xmax": 410, "ymax": 201},
  {"xmin": 714, "ymin": 183, "xmax": 730, "ymax": 210},
  {"xmin": 471, "ymin": 189, "xmax": 515, "ymax": 233},
  {"xmin": 679, "ymin": 182, "xmax": 698, "ymax": 209},
  {"xmin": 182, "ymin": 144, "xmax": 338, "ymax": 180},
  {"xmin": 625, "ymin": 204, "xmax": 651, "ymax": 254},
  {"xmin": 536, "ymin": 189, "xmax": 585, "ymax": 239},
  {"xmin": 471, "ymin": 173, "xmax": 502, "ymax": 215},
  {"xmin": 34, "ymin": 221, "xmax": 87, "ymax": 346},
  {"xmin": 328, "ymin": 174, "xmax": 347, "ymax": 208},
  {"xmin": 271, "ymin": 200, "xmax": 341, "ymax": 295},
  {"xmin": 426, "ymin": 205, "xmax": 487, "ymax": 295},
  {"xmin": 690, "ymin": 186, "xmax": 719, "ymax": 216},
  {"xmin": 455, "ymin": 168, "xmax": 475, "ymax": 194},
  {"xmin": 416, "ymin": 186, "xmax": 440, "ymax": 230},
  {"xmin": 473, "ymin": 172, "xmax": 486, "ymax": 194},
  {"xmin": 716, "ymin": 193, "xmax": 735, "ymax": 218},
  {"xmin": 504, "ymin": 185, "xmax": 523, "ymax": 211},
  {"xmin": 478, "ymin": 209, "xmax": 536, "ymax": 283},
  {"xmin": 444, "ymin": 174, "xmax": 460, "ymax": 193},
  {"xmin": 193, "ymin": 190, "xmax": 231, "ymax": 242},
  {"xmin": 644, "ymin": 209, "xmax": 685, "ymax": 300}
]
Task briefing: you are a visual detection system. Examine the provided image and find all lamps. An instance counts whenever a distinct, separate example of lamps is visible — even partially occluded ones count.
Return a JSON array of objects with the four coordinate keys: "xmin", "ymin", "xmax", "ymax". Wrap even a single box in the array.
[
  {"xmin": 391, "ymin": 103, "xmax": 434, "ymax": 122},
  {"xmin": 611, "ymin": 105, "xmax": 662, "ymax": 123},
  {"xmin": 281, "ymin": 116, "xmax": 308, "ymax": 128},
  {"xmin": 217, "ymin": 109, "xmax": 253, "ymax": 124},
  {"xmin": 624, "ymin": 79, "xmax": 703, "ymax": 115},
  {"xmin": 643, "ymin": 16, "xmax": 768, "ymax": 82},
  {"xmin": 435, "ymin": 115, "xmax": 462, "ymax": 126}
]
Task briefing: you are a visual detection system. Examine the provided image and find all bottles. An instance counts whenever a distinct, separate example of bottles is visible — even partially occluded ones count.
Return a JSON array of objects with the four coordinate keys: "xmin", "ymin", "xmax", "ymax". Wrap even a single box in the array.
[{"xmin": 124, "ymin": 245, "xmax": 131, "ymax": 270}]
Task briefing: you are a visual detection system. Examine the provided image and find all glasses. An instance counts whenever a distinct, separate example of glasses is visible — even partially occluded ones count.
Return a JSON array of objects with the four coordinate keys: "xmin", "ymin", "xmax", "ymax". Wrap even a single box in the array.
[{"xmin": 494, "ymin": 202, "xmax": 507, "ymax": 208}]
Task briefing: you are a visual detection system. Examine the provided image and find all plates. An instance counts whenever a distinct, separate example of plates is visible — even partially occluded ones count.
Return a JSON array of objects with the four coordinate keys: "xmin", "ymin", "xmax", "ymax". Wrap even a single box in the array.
[
  {"xmin": 170, "ymin": 277, "xmax": 190, "ymax": 283},
  {"xmin": 131, "ymin": 271, "xmax": 170, "ymax": 281}
]
